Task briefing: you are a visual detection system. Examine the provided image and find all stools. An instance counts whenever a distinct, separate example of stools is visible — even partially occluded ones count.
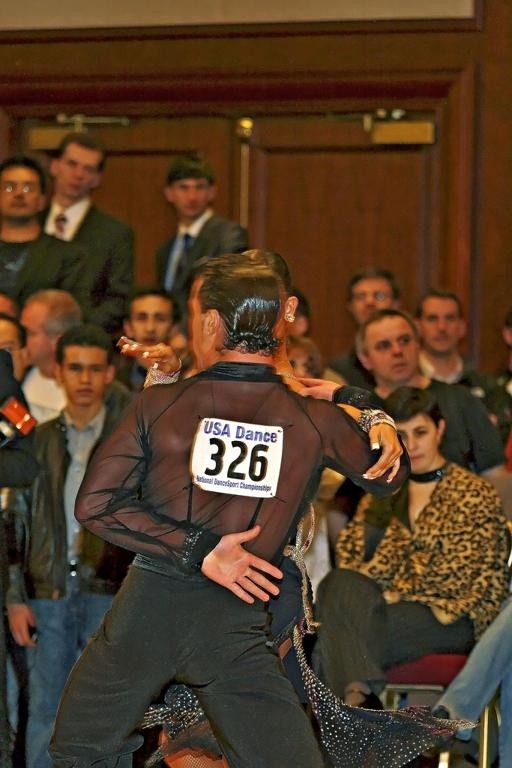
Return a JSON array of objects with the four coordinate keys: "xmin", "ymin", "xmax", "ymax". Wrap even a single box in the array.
[{"xmin": 380, "ymin": 652, "xmax": 500, "ymax": 768}]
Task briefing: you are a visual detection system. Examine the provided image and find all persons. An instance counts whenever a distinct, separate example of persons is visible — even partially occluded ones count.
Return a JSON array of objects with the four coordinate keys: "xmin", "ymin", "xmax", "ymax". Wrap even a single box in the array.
[
  {"xmin": 117, "ymin": 250, "xmax": 476, "ymax": 768},
  {"xmin": 16, "ymin": 289, "xmax": 135, "ymax": 439},
  {"xmin": 310, "ymin": 387, "xmax": 498, "ymax": 716},
  {"xmin": 158, "ymin": 161, "xmax": 249, "ymax": 345},
  {"xmin": 45, "ymin": 256, "xmax": 411, "ymax": 768},
  {"xmin": 355, "ymin": 311, "xmax": 511, "ymax": 525},
  {"xmin": 0, "ymin": 318, "xmax": 65, "ymax": 427},
  {"xmin": 417, "ymin": 593, "xmax": 512, "ymax": 762},
  {"xmin": 0, "ymin": 158, "xmax": 96, "ymax": 321},
  {"xmin": 115, "ymin": 295, "xmax": 186, "ymax": 398},
  {"xmin": 38, "ymin": 134, "xmax": 136, "ymax": 332},
  {"xmin": 0, "ymin": 326, "xmax": 138, "ymax": 767},
  {"xmin": 324, "ymin": 270, "xmax": 406, "ymax": 398},
  {"xmin": 410, "ymin": 292, "xmax": 508, "ymax": 432}
]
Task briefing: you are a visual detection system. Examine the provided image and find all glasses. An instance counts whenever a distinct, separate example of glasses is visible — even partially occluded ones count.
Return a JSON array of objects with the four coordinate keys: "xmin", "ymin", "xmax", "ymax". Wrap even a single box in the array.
[
  {"xmin": 0, "ymin": 181, "xmax": 41, "ymax": 193},
  {"xmin": 350, "ymin": 292, "xmax": 393, "ymax": 300}
]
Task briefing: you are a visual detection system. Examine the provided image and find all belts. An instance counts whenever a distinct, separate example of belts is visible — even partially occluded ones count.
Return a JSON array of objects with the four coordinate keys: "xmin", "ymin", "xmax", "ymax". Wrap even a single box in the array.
[{"xmin": 67, "ymin": 561, "xmax": 80, "ymax": 577}]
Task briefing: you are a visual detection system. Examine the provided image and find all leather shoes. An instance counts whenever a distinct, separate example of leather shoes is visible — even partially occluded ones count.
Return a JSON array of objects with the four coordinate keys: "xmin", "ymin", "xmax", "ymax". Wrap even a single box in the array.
[{"xmin": 344, "ymin": 690, "xmax": 384, "ymax": 712}]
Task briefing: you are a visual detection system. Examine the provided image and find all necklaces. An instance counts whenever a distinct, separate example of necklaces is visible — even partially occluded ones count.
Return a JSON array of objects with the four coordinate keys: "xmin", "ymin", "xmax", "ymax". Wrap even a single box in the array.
[{"xmin": 409, "ymin": 463, "xmax": 448, "ymax": 482}]
